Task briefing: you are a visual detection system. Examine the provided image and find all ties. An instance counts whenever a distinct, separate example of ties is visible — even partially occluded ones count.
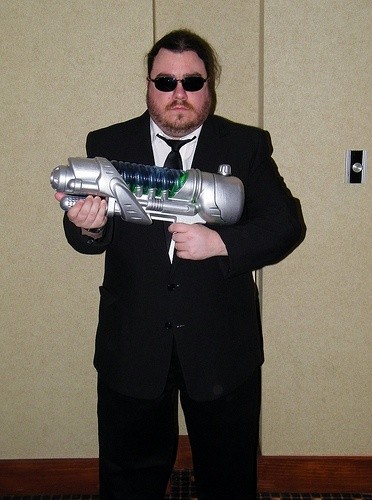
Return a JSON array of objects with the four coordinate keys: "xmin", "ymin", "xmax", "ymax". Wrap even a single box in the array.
[{"xmin": 156, "ymin": 134, "xmax": 196, "ymax": 251}]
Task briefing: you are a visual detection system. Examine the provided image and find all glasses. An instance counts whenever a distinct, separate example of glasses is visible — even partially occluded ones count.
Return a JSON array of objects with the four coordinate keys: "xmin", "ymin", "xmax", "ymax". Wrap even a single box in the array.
[{"xmin": 147, "ymin": 76, "xmax": 211, "ymax": 92}]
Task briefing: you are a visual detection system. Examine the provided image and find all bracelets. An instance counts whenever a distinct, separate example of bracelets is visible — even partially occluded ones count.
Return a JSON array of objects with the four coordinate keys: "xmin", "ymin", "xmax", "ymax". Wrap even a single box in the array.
[{"xmin": 83, "ymin": 228, "xmax": 103, "ymax": 233}]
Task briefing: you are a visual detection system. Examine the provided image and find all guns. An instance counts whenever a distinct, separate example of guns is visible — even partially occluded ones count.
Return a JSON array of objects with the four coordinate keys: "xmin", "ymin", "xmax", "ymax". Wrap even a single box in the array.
[{"xmin": 47, "ymin": 155, "xmax": 246, "ymax": 249}]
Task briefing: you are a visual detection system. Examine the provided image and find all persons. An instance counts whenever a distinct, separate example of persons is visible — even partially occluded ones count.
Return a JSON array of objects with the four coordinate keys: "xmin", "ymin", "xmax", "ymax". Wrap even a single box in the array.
[{"xmin": 55, "ymin": 28, "xmax": 306, "ymax": 500}]
